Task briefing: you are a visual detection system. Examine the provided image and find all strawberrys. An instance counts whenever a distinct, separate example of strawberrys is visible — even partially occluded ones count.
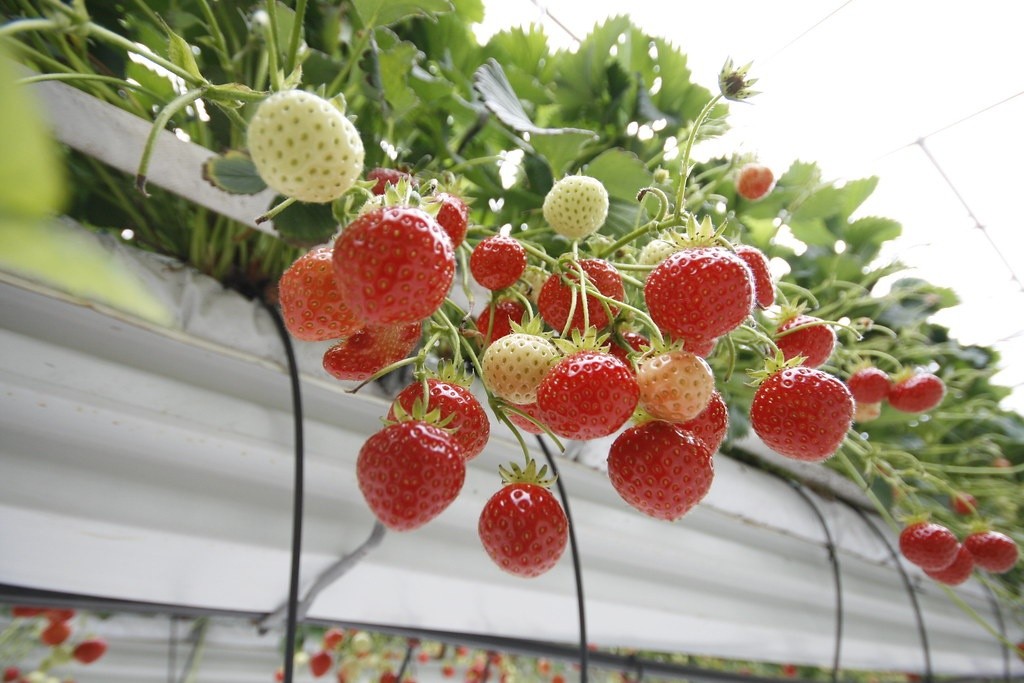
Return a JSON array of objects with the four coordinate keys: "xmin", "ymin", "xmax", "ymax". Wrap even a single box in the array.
[{"xmin": 0, "ymin": 88, "xmax": 1018, "ymax": 683}]
